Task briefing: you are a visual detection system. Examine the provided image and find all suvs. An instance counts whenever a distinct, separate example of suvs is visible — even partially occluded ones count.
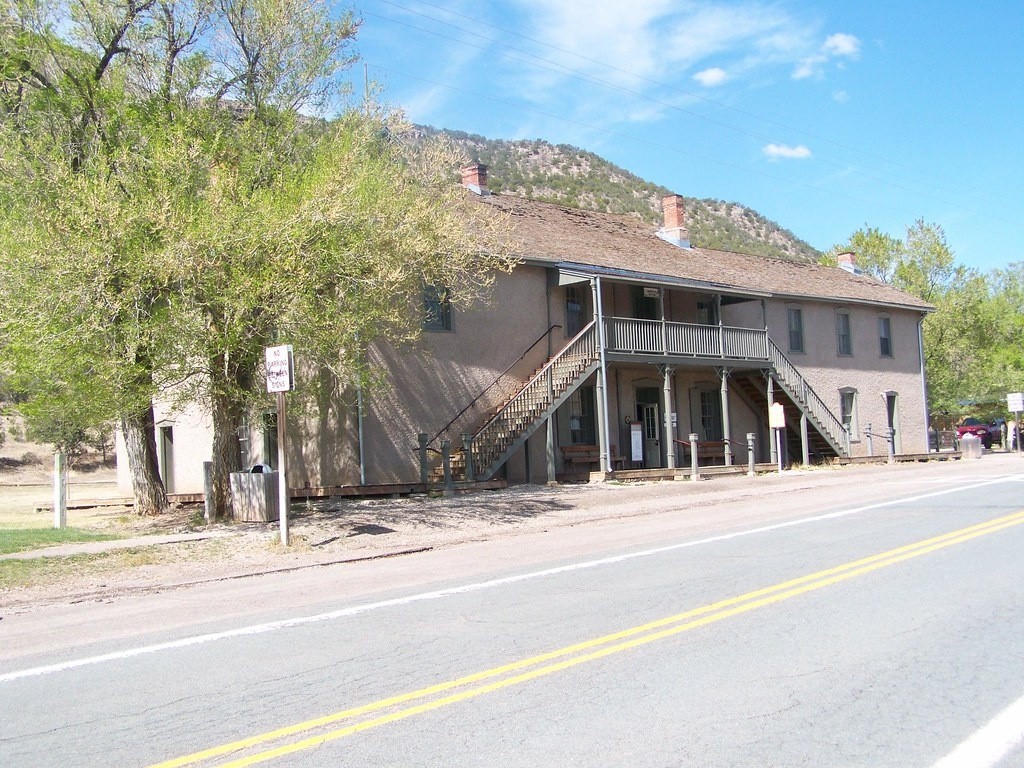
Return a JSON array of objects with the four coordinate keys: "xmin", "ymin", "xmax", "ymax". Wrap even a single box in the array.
[{"xmin": 953, "ymin": 415, "xmax": 1008, "ymax": 450}]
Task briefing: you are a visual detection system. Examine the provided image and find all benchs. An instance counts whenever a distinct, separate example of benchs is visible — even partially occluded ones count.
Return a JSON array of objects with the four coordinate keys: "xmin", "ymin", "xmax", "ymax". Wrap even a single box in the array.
[
  {"xmin": 559, "ymin": 445, "xmax": 625, "ymax": 473},
  {"xmin": 684, "ymin": 442, "xmax": 734, "ymax": 466}
]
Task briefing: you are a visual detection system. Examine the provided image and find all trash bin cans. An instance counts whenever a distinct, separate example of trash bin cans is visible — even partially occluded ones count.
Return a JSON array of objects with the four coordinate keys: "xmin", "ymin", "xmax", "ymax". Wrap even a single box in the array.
[
  {"xmin": 229, "ymin": 467, "xmax": 281, "ymax": 523},
  {"xmin": 960, "ymin": 437, "xmax": 982, "ymax": 459}
]
